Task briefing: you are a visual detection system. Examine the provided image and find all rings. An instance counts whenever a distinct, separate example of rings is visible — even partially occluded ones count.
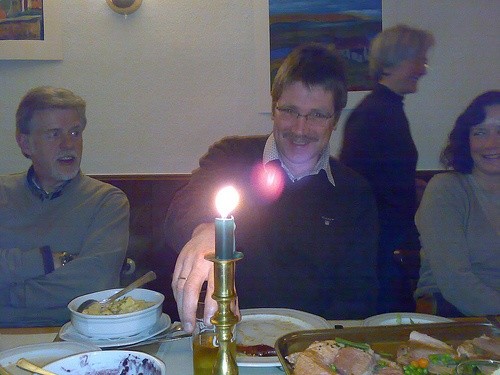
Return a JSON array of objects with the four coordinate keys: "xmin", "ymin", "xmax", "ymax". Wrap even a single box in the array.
[{"xmin": 179, "ymin": 277, "xmax": 188, "ymax": 281}]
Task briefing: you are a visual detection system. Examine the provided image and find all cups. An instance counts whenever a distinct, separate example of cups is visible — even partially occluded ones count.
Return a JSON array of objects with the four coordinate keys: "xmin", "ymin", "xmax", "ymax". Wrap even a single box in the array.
[{"xmin": 192, "ymin": 324, "xmax": 237, "ymax": 375}]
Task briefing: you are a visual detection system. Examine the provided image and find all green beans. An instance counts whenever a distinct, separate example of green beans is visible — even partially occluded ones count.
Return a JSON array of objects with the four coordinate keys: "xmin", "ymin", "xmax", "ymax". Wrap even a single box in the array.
[{"xmin": 331, "ymin": 354, "xmax": 478, "ymax": 375}]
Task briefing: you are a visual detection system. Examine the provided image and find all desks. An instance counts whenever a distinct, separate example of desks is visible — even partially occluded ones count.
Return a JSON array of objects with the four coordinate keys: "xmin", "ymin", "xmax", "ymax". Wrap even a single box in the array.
[{"xmin": 0, "ymin": 317, "xmax": 492, "ymax": 375}]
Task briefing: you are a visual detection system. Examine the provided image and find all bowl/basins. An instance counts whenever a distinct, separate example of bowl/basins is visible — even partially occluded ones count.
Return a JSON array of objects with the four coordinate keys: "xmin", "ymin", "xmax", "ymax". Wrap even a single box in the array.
[{"xmin": 67, "ymin": 288, "xmax": 165, "ymax": 340}]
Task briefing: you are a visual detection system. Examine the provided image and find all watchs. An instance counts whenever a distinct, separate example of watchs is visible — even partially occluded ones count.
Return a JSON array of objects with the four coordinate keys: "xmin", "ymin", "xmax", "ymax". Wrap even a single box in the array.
[{"xmin": 62, "ymin": 252, "xmax": 73, "ymax": 266}]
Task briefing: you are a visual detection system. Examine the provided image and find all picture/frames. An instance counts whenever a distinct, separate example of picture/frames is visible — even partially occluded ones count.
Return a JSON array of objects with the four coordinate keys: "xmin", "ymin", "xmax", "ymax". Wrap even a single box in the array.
[
  {"xmin": 253, "ymin": 0, "xmax": 398, "ymax": 112},
  {"xmin": 0, "ymin": 0, "xmax": 65, "ymax": 61}
]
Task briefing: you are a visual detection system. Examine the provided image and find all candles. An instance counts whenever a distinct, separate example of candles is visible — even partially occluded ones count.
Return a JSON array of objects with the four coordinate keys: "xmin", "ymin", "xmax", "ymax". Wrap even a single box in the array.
[{"xmin": 214, "ymin": 183, "xmax": 241, "ymax": 259}]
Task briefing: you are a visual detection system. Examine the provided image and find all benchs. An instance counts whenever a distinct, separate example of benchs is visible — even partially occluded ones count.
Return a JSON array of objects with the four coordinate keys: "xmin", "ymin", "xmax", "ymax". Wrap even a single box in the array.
[{"xmin": 87, "ymin": 174, "xmax": 192, "ymax": 288}]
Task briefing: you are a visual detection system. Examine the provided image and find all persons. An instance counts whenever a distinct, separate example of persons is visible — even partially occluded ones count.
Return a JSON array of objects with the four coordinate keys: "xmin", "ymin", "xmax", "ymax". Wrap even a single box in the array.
[
  {"xmin": 339, "ymin": 25, "xmax": 434, "ymax": 313},
  {"xmin": 164, "ymin": 45, "xmax": 380, "ymax": 334},
  {"xmin": 415, "ymin": 89, "xmax": 500, "ymax": 319},
  {"xmin": 0, "ymin": 84, "xmax": 130, "ymax": 329}
]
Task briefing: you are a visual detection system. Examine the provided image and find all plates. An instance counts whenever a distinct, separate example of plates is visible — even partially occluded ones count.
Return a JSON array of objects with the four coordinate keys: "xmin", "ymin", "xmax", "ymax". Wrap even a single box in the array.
[
  {"xmin": 59, "ymin": 313, "xmax": 171, "ymax": 346},
  {"xmin": 233, "ymin": 307, "xmax": 334, "ymax": 368},
  {"xmin": 357, "ymin": 312, "xmax": 455, "ymax": 327},
  {"xmin": 32, "ymin": 349, "xmax": 166, "ymax": 375},
  {"xmin": 0, "ymin": 341, "xmax": 102, "ymax": 375}
]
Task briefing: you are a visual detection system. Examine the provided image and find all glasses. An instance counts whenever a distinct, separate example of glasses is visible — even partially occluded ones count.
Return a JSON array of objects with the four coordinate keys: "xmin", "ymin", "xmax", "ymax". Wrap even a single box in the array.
[{"xmin": 275, "ymin": 105, "xmax": 336, "ymax": 123}]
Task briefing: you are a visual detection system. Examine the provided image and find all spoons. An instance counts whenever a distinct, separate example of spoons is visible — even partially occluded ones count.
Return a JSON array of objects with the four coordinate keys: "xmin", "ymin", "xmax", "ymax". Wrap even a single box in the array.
[{"xmin": 76, "ymin": 271, "xmax": 156, "ymax": 312}]
